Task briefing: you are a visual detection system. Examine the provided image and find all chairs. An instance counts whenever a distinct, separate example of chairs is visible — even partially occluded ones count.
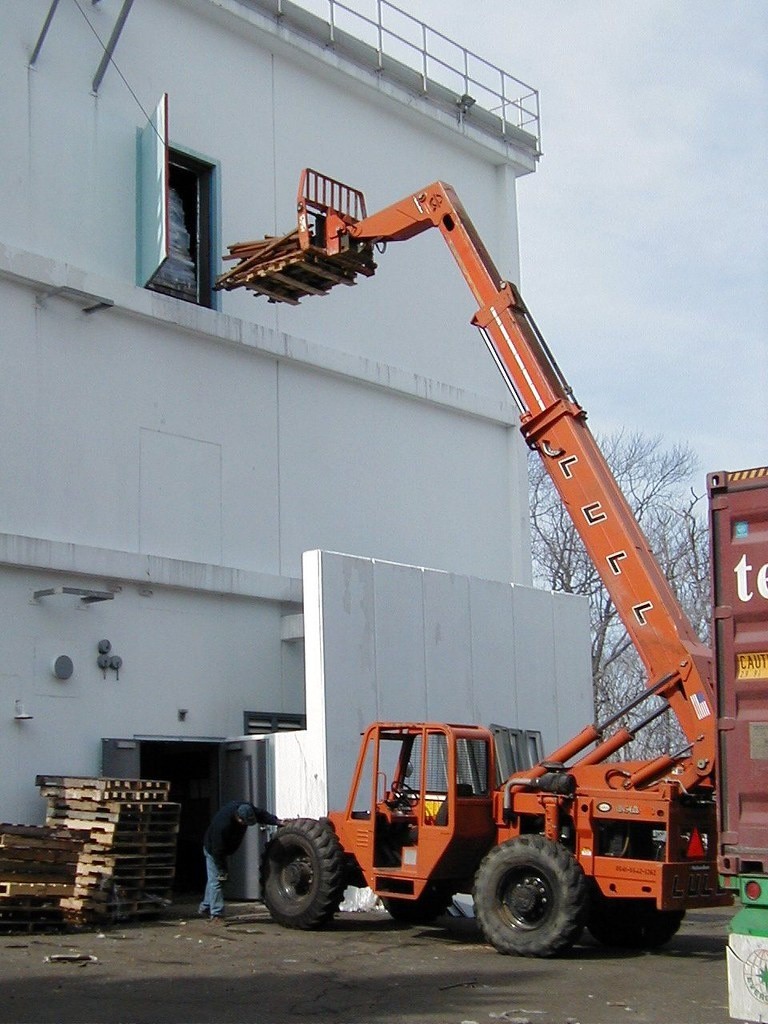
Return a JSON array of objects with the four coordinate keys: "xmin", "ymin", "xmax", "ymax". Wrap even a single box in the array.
[{"xmin": 435, "ymin": 784, "xmax": 473, "ymax": 826}]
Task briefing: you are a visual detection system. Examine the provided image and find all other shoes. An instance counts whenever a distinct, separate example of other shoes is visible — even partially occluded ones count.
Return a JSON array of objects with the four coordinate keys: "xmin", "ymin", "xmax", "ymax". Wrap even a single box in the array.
[{"xmin": 212, "ymin": 916, "xmax": 223, "ymax": 924}]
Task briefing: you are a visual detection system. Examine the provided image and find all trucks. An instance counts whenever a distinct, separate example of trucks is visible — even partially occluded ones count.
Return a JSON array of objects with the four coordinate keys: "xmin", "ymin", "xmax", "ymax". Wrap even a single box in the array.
[{"xmin": 704, "ymin": 466, "xmax": 768, "ymax": 939}]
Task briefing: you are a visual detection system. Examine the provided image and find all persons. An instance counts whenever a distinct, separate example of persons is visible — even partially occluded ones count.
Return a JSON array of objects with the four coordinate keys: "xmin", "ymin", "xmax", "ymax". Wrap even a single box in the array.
[{"xmin": 197, "ymin": 801, "xmax": 293, "ymax": 928}]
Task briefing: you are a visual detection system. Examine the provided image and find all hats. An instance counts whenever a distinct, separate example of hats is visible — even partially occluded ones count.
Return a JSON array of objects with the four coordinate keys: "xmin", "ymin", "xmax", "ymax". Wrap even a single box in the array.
[{"xmin": 237, "ymin": 804, "xmax": 258, "ymax": 826}]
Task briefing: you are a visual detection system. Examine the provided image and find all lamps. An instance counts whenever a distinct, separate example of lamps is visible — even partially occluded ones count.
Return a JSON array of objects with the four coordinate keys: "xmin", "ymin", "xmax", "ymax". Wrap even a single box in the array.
[
  {"xmin": 458, "ymin": 94, "xmax": 477, "ymax": 110},
  {"xmin": 13, "ymin": 700, "xmax": 34, "ymax": 720}
]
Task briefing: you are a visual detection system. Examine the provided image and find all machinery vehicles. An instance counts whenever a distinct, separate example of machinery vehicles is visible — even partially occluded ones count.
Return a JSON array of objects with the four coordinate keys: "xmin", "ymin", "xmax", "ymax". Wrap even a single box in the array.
[{"xmin": 222, "ymin": 176, "xmax": 716, "ymax": 964}]
